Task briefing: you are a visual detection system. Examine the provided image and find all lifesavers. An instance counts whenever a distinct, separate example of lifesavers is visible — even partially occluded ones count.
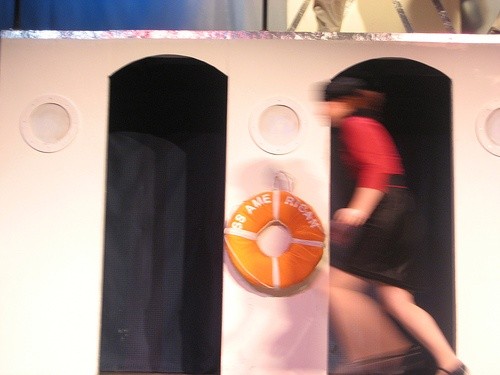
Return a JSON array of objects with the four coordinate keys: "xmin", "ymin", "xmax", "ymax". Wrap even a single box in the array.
[{"xmin": 224, "ymin": 191, "xmax": 327, "ymax": 289}]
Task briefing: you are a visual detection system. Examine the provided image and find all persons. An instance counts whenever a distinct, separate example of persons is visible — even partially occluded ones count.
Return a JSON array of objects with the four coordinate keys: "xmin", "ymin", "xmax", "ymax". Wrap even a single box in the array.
[{"xmin": 313, "ymin": 77, "xmax": 466, "ymax": 374}]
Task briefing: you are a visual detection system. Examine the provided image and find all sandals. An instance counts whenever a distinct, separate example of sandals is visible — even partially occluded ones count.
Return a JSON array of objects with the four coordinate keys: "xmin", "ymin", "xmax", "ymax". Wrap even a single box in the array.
[{"xmin": 436, "ymin": 361, "xmax": 467, "ymax": 374}]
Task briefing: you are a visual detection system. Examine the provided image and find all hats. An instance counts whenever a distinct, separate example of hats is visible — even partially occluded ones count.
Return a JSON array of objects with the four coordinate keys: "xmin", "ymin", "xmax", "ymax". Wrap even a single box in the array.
[{"xmin": 323, "ymin": 77, "xmax": 383, "ymax": 100}]
